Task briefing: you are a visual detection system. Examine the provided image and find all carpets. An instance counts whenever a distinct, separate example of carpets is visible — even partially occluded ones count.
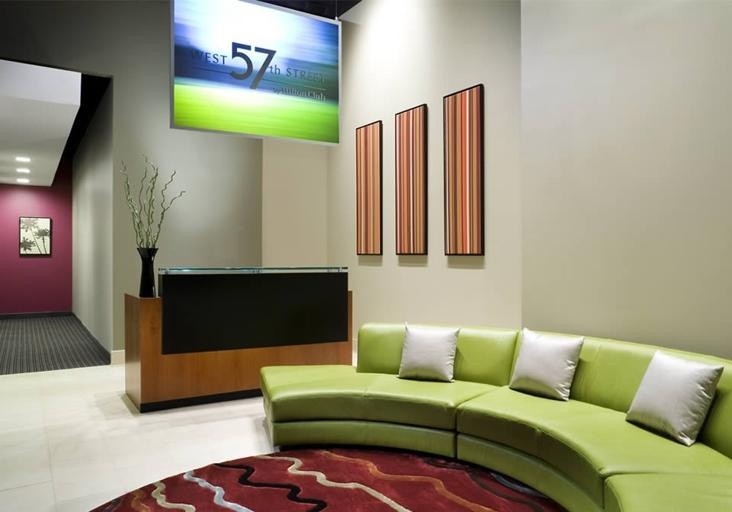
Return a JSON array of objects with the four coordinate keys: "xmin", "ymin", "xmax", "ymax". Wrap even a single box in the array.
[{"xmin": 87, "ymin": 449, "xmax": 567, "ymax": 511}]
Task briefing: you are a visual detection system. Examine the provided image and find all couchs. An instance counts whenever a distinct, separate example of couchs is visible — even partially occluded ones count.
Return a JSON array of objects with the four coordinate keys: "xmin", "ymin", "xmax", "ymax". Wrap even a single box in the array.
[{"xmin": 259, "ymin": 323, "xmax": 732, "ymax": 511}]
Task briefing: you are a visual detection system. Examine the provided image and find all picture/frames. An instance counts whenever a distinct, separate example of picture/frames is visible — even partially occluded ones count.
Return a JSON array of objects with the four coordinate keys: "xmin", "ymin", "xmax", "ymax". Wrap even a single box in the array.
[{"xmin": 18, "ymin": 216, "xmax": 52, "ymax": 257}]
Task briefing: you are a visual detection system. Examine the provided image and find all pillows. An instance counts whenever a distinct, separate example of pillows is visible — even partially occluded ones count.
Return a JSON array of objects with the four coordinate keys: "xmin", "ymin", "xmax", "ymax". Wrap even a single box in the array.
[
  {"xmin": 625, "ymin": 349, "xmax": 724, "ymax": 448},
  {"xmin": 396, "ymin": 325, "xmax": 461, "ymax": 382},
  {"xmin": 510, "ymin": 329, "xmax": 584, "ymax": 400}
]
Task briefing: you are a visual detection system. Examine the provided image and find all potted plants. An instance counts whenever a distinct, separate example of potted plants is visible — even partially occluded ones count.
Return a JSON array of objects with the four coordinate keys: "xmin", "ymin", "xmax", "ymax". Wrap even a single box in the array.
[{"xmin": 119, "ymin": 156, "xmax": 186, "ymax": 297}]
